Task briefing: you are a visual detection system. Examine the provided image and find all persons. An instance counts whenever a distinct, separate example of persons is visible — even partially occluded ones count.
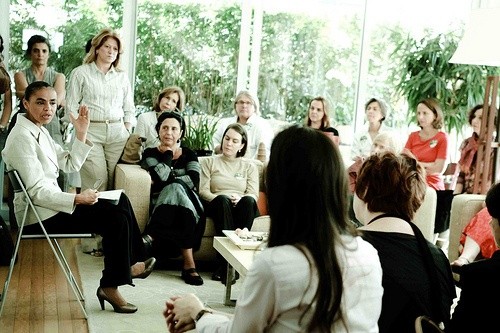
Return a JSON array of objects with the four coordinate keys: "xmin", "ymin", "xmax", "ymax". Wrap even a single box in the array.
[
  {"xmin": 163, "ymin": 125, "xmax": 384, "ymax": 333},
  {"xmin": 66, "ymin": 28, "xmax": 135, "ymax": 257},
  {"xmin": 352, "ymin": 151, "xmax": 457, "ymax": 333},
  {"xmin": 351, "ymin": 97, "xmax": 396, "ymax": 161},
  {"xmin": 369, "ymin": 134, "xmax": 395, "ymax": 155},
  {"xmin": 306, "ymin": 96, "xmax": 338, "ymax": 136},
  {"xmin": 450, "ymin": 203, "xmax": 500, "ymax": 266},
  {"xmin": 135, "ymin": 86, "xmax": 186, "ymax": 149},
  {"xmin": 3, "ymin": 36, "xmax": 65, "ymax": 232},
  {"xmin": 140, "ymin": 112, "xmax": 207, "ymax": 285},
  {"xmin": 434, "ymin": 105, "xmax": 498, "ymax": 247},
  {"xmin": 401, "ymin": 97, "xmax": 450, "ymax": 190},
  {"xmin": 199, "ymin": 125, "xmax": 258, "ymax": 285},
  {"xmin": 445, "ymin": 183, "xmax": 500, "ymax": 333},
  {"xmin": 1, "ymin": 81, "xmax": 156, "ymax": 314},
  {"xmin": 212, "ymin": 89, "xmax": 266, "ymax": 162},
  {"xmin": 0, "ymin": 36, "xmax": 13, "ymax": 164}
]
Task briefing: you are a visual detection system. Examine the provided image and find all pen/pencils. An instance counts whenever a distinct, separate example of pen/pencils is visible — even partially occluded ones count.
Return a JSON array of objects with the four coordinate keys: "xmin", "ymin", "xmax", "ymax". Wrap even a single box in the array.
[{"xmin": 96, "ymin": 181, "xmax": 104, "ymax": 193}]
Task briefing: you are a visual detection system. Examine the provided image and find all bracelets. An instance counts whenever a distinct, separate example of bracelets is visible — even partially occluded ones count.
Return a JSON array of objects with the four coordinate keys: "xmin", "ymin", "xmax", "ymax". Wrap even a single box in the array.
[{"xmin": 196, "ymin": 310, "xmax": 212, "ymax": 321}]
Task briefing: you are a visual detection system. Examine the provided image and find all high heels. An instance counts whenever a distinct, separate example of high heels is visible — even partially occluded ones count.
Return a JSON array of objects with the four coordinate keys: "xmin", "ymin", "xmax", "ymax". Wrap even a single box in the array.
[
  {"xmin": 128, "ymin": 257, "xmax": 157, "ymax": 287},
  {"xmin": 97, "ymin": 286, "xmax": 137, "ymax": 313}
]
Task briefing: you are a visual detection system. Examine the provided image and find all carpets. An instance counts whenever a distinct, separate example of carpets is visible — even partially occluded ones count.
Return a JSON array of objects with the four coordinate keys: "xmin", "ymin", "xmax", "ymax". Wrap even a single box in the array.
[{"xmin": 72, "ymin": 241, "xmax": 240, "ymax": 333}]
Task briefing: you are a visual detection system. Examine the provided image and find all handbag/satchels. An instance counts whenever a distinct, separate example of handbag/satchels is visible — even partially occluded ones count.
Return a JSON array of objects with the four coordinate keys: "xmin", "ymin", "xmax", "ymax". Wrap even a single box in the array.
[{"xmin": 0, "ymin": 215, "xmax": 18, "ymax": 266}]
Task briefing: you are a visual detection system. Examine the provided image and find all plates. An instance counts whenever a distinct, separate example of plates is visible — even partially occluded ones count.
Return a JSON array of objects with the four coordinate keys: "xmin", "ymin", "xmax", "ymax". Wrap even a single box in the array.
[{"xmin": 222, "ymin": 229, "xmax": 269, "ymax": 250}]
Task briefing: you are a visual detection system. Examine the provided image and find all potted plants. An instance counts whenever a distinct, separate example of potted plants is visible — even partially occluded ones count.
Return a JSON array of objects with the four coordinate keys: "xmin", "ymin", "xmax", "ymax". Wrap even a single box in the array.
[{"xmin": 182, "ymin": 110, "xmax": 223, "ymax": 156}]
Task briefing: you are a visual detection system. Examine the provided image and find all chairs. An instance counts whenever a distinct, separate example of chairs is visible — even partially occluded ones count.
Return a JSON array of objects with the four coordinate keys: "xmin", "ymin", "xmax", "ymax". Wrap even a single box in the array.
[
  {"xmin": 0, "ymin": 167, "xmax": 92, "ymax": 318},
  {"xmin": 450, "ymin": 194, "xmax": 496, "ymax": 284}
]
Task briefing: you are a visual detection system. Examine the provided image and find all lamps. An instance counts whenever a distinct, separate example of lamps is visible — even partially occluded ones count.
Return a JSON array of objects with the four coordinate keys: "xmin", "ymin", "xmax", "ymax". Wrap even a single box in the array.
[{"xmin": 448, "ymin": 0, "xmax": 500, "ymax": 194}]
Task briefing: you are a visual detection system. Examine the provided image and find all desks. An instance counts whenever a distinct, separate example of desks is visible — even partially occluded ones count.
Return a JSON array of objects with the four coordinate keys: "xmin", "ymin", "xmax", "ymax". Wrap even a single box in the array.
[{"xmin": 214, "ymin": 236, "xmax": 259, "ymax": 306}]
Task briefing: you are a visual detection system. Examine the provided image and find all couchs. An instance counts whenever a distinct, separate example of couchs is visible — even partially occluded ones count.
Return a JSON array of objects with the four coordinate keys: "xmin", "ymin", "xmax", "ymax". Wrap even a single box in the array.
[{"xmin": 113, "ymin": 158, "xmax": 217, "ymax": 258}]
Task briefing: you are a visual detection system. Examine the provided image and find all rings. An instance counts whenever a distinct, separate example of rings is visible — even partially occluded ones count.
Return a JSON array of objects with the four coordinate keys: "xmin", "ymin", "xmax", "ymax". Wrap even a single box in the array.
[{"xmin": 84, "ymin": 115, "xmax": 86, "ymax": 118}]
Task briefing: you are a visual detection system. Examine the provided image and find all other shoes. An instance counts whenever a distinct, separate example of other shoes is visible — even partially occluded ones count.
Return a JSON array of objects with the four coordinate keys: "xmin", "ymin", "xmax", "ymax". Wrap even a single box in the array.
[
  {"xmin": 182, "ymin": 268, "xmax": 203, "ymax": 285},
  {"xmin": 451, "ymin": 257, "xmax": 471, "ymax": 273},
  {"xmin": 142, "ymin": 235, "xmax": 156, "ymax": 249}
]
